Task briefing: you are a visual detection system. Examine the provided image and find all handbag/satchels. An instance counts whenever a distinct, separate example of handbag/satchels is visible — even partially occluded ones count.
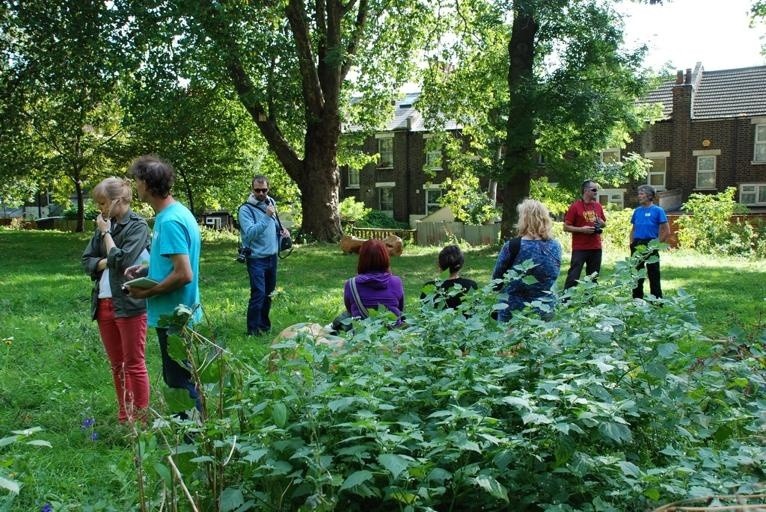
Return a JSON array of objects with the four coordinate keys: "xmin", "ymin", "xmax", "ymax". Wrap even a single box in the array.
[{"xmin": 279, "ymin": 234, "xmax": 292, "ymax": 251}]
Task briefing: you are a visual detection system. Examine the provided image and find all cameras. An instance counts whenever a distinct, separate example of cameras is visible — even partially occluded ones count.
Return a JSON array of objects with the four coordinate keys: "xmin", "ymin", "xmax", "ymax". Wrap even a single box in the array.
[
  {"xmin": 594, "ymin": 221, "xmax": 602, "ymax": 234},
  {"xmin": 237, "ymin": 247, "xmax": 251, "ymax": 263}
]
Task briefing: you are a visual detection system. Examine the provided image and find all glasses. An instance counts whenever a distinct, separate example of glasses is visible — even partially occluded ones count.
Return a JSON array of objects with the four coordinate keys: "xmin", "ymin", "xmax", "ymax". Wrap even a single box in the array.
[
  {"xmin": 254, "ymin": 188, "xmax": 269, "ymax": 193},
  {"xmin": 591, "ymin": 188, "xmax": 598, "ymax": 192}
]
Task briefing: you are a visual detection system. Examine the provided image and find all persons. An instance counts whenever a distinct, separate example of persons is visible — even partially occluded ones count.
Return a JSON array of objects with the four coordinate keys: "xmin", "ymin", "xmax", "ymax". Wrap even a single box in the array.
[
  {"xmin": 323, "ymin": 197, "xmax": 562, "ymax": 338},
  {"xmin": 81, "ymin": 153, "xmax": 205, "ymax": 453},
  {"xmin": 560, "ymin": 180, "xmax": 670, "ymax": 309},
  {"xmin": 235, "ymin": 175, "xmax": 293, "ymax": 339}
]
